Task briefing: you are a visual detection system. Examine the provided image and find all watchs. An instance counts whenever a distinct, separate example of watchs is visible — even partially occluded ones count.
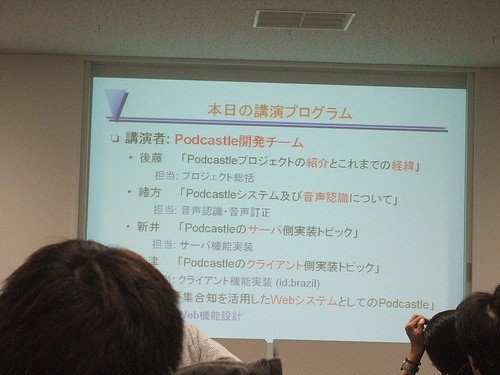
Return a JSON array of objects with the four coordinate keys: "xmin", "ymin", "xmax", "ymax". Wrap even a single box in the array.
[{"xmin": 401, "ymin": 360, "xmax": 420, "ymax": 373}]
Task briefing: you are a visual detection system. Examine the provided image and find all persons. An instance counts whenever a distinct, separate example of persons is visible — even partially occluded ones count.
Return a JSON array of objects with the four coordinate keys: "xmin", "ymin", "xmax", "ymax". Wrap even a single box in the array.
[
  {"xmin": 178, "ymin": 321, "xmax": 245, "ymax": 369},
  {"xmin": 0, "ymin": 233, "xmax": 184, "ymax": 374},
  {"xmin": 454, "ymin": 291, "xmax": 500, "ymax": 375},
  {"xmin": 400, "ymin": 311, "xmax": 473, "ymax": 375}
]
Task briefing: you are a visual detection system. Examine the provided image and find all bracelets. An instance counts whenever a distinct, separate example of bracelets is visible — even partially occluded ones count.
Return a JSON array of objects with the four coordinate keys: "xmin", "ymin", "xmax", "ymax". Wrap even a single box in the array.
[{"xmin": 405, "ymin": 357, "xmax": 422, "ymax": 365}]
